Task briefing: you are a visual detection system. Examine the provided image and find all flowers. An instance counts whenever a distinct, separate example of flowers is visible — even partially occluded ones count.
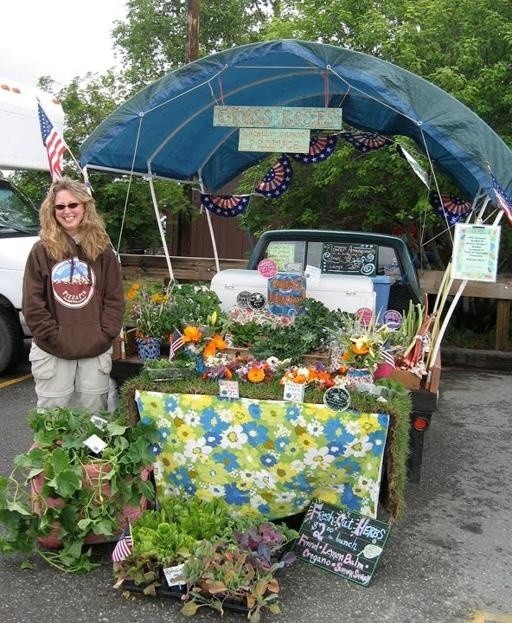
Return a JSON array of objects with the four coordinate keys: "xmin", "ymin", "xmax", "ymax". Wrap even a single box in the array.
[{"xmin": 126, "ymin": 281, "xmax": 439, "ymax": 394}]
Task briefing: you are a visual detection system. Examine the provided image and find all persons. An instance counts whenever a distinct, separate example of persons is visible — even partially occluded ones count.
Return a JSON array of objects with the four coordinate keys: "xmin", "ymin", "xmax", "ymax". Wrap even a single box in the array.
[{"xmin": 20, "ymin": 178, "xmax": 125, "ymax": 415}]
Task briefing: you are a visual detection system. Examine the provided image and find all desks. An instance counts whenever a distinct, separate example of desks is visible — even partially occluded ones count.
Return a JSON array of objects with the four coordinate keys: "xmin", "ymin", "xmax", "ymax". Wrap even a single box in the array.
[{"xmin": 120, "ymin": 359, "xmax": 411, "ymax": 533}]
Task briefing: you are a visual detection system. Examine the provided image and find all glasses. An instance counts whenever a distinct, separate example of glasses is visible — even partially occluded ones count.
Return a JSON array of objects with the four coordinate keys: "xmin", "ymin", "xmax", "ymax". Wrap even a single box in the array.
[{"xmin": 53, "ymin": 203, "xmax": 82, "ymax": 209}]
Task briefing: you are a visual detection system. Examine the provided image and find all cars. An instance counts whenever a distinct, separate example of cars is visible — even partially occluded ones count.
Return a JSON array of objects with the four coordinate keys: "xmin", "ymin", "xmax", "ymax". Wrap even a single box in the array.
[{"xmin": 0, "ymin": 178, "xmax": 48, "ymax": 376}]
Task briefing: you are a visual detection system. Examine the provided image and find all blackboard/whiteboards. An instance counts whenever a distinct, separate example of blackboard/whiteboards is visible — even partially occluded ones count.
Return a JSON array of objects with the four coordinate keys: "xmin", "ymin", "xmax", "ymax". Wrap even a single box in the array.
[{"xmin": 293, "ymin": 497, "xmax": 389, "ymax": 587}]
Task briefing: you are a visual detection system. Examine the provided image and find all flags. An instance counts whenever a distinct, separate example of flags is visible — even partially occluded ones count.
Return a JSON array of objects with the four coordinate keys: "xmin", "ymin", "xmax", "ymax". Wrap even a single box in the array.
[
  {"xmin": 36, "ymin": 101, "xmax": 67, "ymax": 179},
  {"xmin": 111, "ymin": 522, "xmax": 134, "ymax": 562}
]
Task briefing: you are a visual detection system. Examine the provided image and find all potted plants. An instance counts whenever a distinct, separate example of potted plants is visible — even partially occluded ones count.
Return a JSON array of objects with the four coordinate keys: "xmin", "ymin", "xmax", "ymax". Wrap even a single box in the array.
[{"xmin": 0, "ymin": 407, "xmax": 301, "ymax": 623}]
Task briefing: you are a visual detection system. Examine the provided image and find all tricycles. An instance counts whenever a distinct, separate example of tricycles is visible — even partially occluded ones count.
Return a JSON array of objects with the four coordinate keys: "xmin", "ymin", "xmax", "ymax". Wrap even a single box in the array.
[{"xmin": 244, "ymin": 226, "xmax": 439, "ymax": 487}]
